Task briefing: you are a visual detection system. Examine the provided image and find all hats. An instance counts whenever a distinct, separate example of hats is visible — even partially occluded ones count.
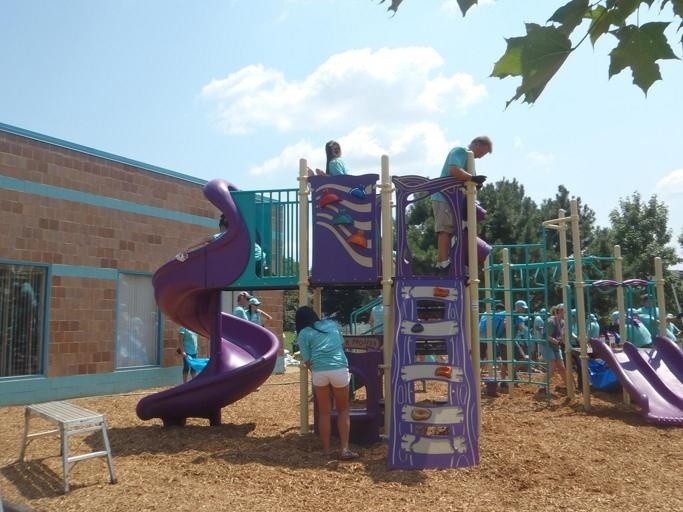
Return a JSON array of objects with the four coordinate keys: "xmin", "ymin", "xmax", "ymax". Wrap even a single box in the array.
[
  {"xmin": 247, "ymin": 297, "xmax": 261, "ymax": 307},
  {"xmin": 514, "ymin": 300, "xmax": 528, "ymax": 309},
  {"xmin": 555, "ymin": 303, "xmax": 564, "ymax": 310},
  {"xmin": 587, "ymin": 313, "xmax": 597, "ymax": 322},
  {"xmin": 610, "ymin": 311, "xmax": 619, "ymax": 326}
]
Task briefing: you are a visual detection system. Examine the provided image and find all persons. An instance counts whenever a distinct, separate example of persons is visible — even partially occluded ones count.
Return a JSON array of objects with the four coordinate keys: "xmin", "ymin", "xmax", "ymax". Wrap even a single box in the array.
[
  {"xmin": 367, "ymin": 294, "xmax": 384, "ymax": 336},
  {"xmin": 234, "ymin": 292, "xmax": 273, "ymax": 322},
  {"xmin": 177, "ymin": 213, "xmax": 268, "ymax": 278},
  {"xmin": 246, "ymin": 296, "xmax": 260, "ymax": 325},
  {"xmin": 477, "ymin": 291, "xmax": 683, "ymax": 393},
  {"xmin": 316, "ymin": 140, "xmax": 347, "ymax": 176},
  {"xmin": 293, "ymin": 306, "xmax": 359, "ymax": 460},
  {"xmin": 177, "ymin": 327, "xmax": 198, "ymax": 383},
  {"xmin": 430, "ymin": 135, "xmax": 492, "ymax": 273}
]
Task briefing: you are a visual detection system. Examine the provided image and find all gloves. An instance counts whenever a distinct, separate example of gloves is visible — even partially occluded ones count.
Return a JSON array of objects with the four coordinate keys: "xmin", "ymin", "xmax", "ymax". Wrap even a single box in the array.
[
  {"xmin": 476, "ymin": 184, "xmax": 483, "ymax": 190},
  {"xmin": 470, "ymin": 175, "xmax": 487, "ymax": 184}
]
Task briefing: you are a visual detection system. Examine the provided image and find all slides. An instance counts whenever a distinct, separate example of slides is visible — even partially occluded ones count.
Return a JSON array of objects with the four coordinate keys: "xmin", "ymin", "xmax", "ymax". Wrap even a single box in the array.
[
  {"xmin": 590, "ymin": 335, "xmax": 681, "ymax": 428},
  {"xmin": 134, "ymin": 178, "xmax": 277, "ymax": 427}
]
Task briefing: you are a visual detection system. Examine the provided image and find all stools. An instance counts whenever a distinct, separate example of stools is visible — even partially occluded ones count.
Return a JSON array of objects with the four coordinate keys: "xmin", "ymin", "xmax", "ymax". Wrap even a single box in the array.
[{"xmin": 19, "ymin": 399, "xmax": 117, "ymax": 494}]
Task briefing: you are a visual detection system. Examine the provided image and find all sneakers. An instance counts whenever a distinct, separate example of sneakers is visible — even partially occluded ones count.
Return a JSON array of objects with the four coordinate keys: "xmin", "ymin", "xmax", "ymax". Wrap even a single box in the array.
[
  {"xmin": 434, "ymin": 262, "xmax": 451, "ymax": 276},
  {"xmin": 495, "ymin": 374, "xmax": 580, "ymax": 396},
  {"xmin": 313, "ymin": 446, "xmax": 360, "ymax": 460}
]
